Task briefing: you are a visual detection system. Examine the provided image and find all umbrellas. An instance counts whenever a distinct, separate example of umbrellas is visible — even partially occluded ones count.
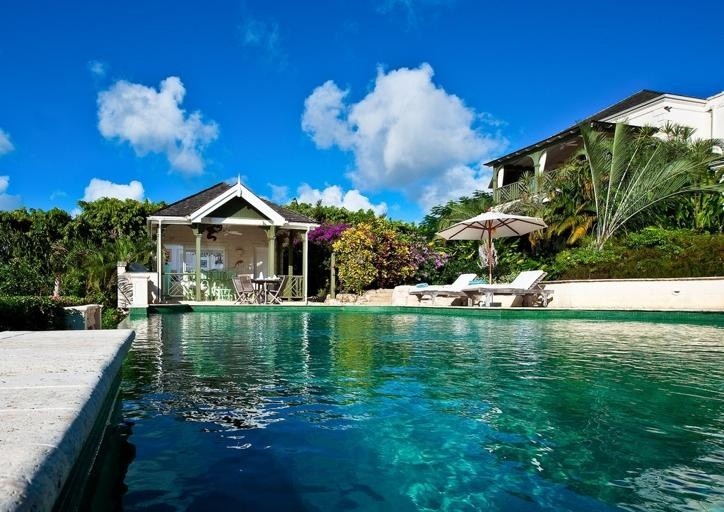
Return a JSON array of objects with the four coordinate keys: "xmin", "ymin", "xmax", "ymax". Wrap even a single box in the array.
[{"xmin": 434, "ymin": 208, "xmax": 548, "ymax": 282}]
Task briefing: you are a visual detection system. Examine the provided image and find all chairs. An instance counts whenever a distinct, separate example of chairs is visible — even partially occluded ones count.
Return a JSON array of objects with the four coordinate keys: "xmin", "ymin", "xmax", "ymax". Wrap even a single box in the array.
[
  {"xmin": 409, "ymin": 273, "xmax": 477, "ymax": 307},
  {"xmin": 264, "ymin": 274, "xmax": 285, "ymax": 304},
  {"xmin": 468, "ymin": 271, "xmax": 547, "ymax": 309},
  {"xmin": 231, "ymin": 275, "xmax": 255, "ymax": 304},
  {"xmin": 236, "ymin": 274, "xmax": 261, "ymax": 305}
]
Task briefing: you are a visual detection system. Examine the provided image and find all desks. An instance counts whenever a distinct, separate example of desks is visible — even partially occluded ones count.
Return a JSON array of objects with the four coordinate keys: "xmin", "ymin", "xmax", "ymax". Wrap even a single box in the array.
[{"xmin": 251, "ymin": 277, "xmax": 280, "ymax": 305}]
